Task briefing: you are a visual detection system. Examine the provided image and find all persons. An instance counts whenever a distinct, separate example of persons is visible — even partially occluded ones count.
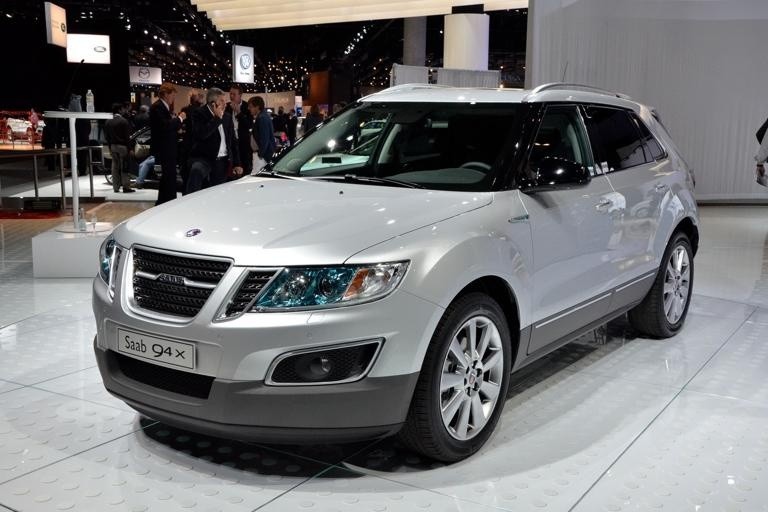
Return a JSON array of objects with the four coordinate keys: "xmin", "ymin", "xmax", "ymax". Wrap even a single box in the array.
[
  {"xmin": 752, "ymin": 117, "xmax": 768, "ymax": 187},
  {"xmin": 303, "ymin": 104, "xmax": 342, "ymax": 132},
  {"xmin": 273, "ymin": 106, "xmax": 297, "ymax": 147},
  {"xmin": 42, "ymin": 118, "xmax": 90, "ymax": 177},
  {"xmin": 150, "ymin": 85, "xmax": 186, "ymax": 206},
  {"xmin": 104, "ymin": 102, "xmax": 135, "ymax": 193},
  {"xmin": 248, "ymin": 96, "xmax": 277, "ymax": 165},
  {"xmin": 180, "ymin": 85, "xmax": 253, "ymax": 192},
  {"xmin": 124, "ymin": 102, "xmax": 155, "ymax": 187}
]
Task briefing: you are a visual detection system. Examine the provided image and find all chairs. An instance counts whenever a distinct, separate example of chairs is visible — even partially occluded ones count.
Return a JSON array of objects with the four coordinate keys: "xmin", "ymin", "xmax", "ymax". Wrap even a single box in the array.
[{"xmin": 466, "ymin": 128, "xmax": 585, "ymax": 181}]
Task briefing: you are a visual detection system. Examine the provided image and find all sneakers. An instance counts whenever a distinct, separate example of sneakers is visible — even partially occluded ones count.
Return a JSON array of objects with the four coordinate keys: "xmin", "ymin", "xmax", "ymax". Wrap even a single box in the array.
[{"xmin": 130, "ymin": 183, "xmax": 142, "ymax": 188}]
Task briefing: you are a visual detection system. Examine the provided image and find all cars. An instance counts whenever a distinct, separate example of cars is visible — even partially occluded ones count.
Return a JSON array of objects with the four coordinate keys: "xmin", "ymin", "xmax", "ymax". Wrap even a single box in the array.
[
  {"xmin": 103, "ymin": 126, "xmax": 151, "ymax": 159},
  {"xmin": 92, "ymin": 82, "xmax": 699, "ymax": 461},
  {"xmin": 6, "ymin": 118, "xmax": 46, "ymax": 143}
]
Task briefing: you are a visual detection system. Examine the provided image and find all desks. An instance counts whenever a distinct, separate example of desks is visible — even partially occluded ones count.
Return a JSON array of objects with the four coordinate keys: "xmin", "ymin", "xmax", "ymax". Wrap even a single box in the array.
[{"xmin": 43, "ymin": 108, "xmax": 117, "ymax": 234}]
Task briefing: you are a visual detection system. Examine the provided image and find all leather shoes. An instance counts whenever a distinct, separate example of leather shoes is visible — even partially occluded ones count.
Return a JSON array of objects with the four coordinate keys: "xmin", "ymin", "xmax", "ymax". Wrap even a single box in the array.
[{"xmin": 123, "ymin": 188, "xmax": 135, "ymax": 193}]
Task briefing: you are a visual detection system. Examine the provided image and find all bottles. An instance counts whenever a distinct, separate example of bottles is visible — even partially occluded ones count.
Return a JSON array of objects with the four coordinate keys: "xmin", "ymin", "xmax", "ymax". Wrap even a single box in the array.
[
  {"xmin": 79, "ymin": 208, "xmax": 86, "ymax": 230},
  {"xmin": 86, "ymin": 90, "xmax": 94, "ymax": 113}
]
